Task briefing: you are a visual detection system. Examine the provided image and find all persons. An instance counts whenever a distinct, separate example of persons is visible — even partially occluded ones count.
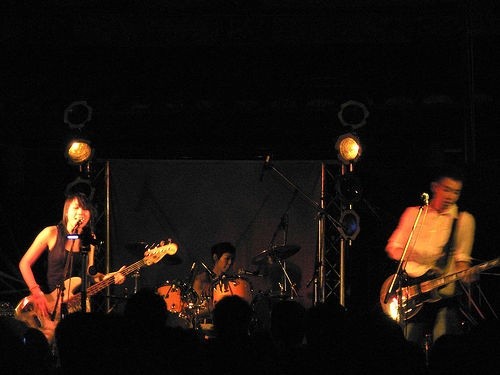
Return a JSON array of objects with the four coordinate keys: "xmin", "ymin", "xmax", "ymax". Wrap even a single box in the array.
[
  {"xmin": 193, "ymin": 241, "xmax": 236, "ymax": 301},
  {"xmin": 1, "ymin": 293, "xmax": 500, "ymax": 375},
  {"xmin": 384, "ymin": 167, "xmax": 475, "ymax": 343},
  {"xmin": 18, "ymin": 192, "xmax": 125, "ymax": 357}
]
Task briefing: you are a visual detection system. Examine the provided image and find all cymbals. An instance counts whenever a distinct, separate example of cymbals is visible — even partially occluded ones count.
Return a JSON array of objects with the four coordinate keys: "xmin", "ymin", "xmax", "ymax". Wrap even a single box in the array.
[
  {"xmin": 267, "ymin": 294, "xmax": 304, "ymax": 300},
  {"xmin": 252, "ymin": 242, "xmax": 302, "ymax": 266}
]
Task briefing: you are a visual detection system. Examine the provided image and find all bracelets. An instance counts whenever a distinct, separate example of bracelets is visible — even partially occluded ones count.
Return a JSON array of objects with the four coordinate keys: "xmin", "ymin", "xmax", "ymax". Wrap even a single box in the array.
[{"xmin": 29, "ymin": 285, "xmax": 39, "ymax": 290}]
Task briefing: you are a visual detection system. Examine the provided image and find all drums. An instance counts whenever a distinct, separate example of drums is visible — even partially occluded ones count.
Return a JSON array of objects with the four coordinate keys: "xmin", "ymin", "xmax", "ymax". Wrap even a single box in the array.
[
  {"xmin": 208, "ymin": 275, "xmax": 255, "ymax": 309},
  {"xmin": 154, "ymin": 282, "xmax": 197, "ymax": 329},
  {"xmin": 192, "ymin": 296, "xmax": 219, "ymax": 339}
]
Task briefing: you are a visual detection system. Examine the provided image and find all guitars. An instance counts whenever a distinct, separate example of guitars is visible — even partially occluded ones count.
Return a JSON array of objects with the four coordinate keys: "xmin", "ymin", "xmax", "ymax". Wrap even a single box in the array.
[
  {"xmin": 13, "ymin": 237, "xmax": 179, "ymax": 345},
  {"xmin": 380, "ymin": 256, "xmax": 500, "ymax": 321}
]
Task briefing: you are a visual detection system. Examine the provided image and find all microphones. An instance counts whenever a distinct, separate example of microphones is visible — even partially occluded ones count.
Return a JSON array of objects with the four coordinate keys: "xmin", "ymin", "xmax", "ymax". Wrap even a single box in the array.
[
  {"xmin": 419, "ymin": 193, "xmax": 430, "ymax": 205},
  {"xmin": 260, "ymin": 156, "xmax": 270, "ymax": 183},
  {"xmin": 238, "ymin": 268, "xmax": 263, "ymax": 277},
  {"xmin": 71, "ymin": 216, "xmax": 86, "ymax": 233}
]
